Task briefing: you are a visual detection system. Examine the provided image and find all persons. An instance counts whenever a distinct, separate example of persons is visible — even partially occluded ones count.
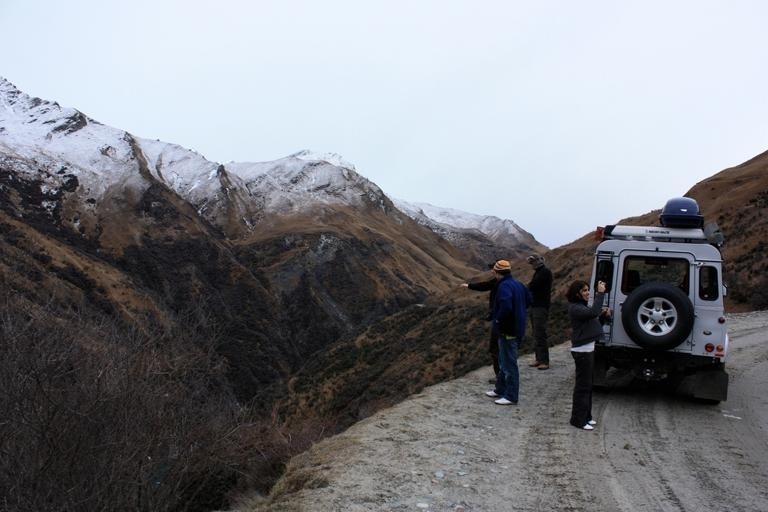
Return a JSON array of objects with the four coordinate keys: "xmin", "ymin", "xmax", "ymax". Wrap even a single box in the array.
[
  {"xmin": 563, "ymin": 279, "xmax": 613, "ymax": 432},
  {"xmin": 456, "ymin": 259, "xmax": 532, "ymax": 406},
  {"xmin": 523, "ymin": 253, "xmax": 552, "ymax": 370}
]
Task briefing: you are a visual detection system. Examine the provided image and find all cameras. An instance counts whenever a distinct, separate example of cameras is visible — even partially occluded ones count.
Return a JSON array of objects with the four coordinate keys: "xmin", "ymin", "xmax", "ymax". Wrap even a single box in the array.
[{"xmin": 598, "ymin": 279, "xmax": 609, "ymax": 284}]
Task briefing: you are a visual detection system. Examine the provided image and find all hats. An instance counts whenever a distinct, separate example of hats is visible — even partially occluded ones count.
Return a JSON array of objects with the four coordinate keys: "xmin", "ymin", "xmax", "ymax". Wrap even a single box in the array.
[
  {"xmin": 488, "ymin": 258, "xmax": 511, "ymax": 271},
  {"xmin": 527, "ymin": 254, "xmax": 544, "ymax": 264}
]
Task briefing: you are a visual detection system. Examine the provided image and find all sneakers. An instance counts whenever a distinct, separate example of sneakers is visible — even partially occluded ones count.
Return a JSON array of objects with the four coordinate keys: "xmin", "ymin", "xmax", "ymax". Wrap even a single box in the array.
[
  {"xmin": 528, "ymin": 361, "xmax": 549, "ymax": 369},
  {"xmin": 486, "ymin": 377, "xmax": 516, "ymax": 405}
]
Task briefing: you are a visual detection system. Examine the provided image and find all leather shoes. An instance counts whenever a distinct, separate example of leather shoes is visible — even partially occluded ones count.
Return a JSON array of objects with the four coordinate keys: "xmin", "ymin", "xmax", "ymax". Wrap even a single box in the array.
[{"xmin": 584, "ymin": 420, "xmax": 596, "ymax": 430}]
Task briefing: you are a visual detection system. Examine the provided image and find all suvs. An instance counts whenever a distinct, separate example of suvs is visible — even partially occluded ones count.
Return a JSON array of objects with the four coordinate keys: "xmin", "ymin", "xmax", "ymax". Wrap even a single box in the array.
[{"xmin": 582, "ymin": 195, "xmax": 729, "ymax": 407}]
